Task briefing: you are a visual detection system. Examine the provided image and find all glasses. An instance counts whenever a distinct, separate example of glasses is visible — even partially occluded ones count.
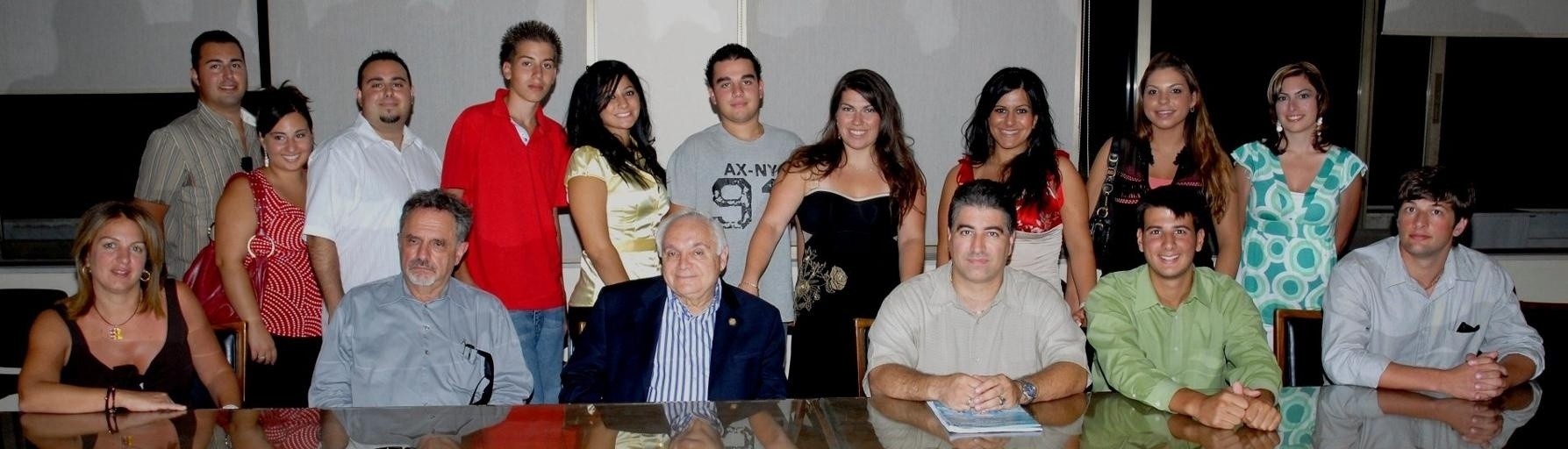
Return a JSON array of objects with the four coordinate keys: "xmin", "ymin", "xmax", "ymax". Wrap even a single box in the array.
[{"xmin": 461, "ymin": 344, "xmax": 497, "ymax": 408}]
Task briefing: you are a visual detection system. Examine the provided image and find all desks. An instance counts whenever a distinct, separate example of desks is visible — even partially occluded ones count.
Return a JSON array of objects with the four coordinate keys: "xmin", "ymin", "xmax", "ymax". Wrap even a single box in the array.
[{"xmin": 1, "ymin": 385, "xmax": 1568, "ymax": 449}]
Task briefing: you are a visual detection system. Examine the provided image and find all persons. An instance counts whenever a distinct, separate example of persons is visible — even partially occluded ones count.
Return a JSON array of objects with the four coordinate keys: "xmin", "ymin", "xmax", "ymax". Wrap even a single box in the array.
[
  {"xmin": 17, "ymin": 383, "xmax": 1553, "ymax": 448},
  {"xmin": 12, "ymin": 16, "xmax": 1546, "ymax": 437}
]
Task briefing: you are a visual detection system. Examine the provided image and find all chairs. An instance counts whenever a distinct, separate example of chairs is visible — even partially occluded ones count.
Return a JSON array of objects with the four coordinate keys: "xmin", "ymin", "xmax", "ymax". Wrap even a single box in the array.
[
  {"xmin": 208, "ymin": 320, "xmax": 246, "ymax": 405},
  {"xmin": 854, "ymin": 318, "xmax": 874, "ymax": 398},
  {"xmin": 1, "ymin": 287, "xmax": 69, "ymax": 398},
  {"xmin": 1274, "ymin": 308, "xmax": 1324, "ymax": 388}
]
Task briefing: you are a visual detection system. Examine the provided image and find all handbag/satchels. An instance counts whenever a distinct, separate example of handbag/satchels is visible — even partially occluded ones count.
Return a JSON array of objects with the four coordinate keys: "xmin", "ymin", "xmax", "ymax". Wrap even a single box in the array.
[
  {"xmin": 177, "ymin": 233, "xmax": 269, "ymax": 332},
  {"xmin": 1085, "ymin": 203, "xmax": 1119, "ymax": 271}
]
